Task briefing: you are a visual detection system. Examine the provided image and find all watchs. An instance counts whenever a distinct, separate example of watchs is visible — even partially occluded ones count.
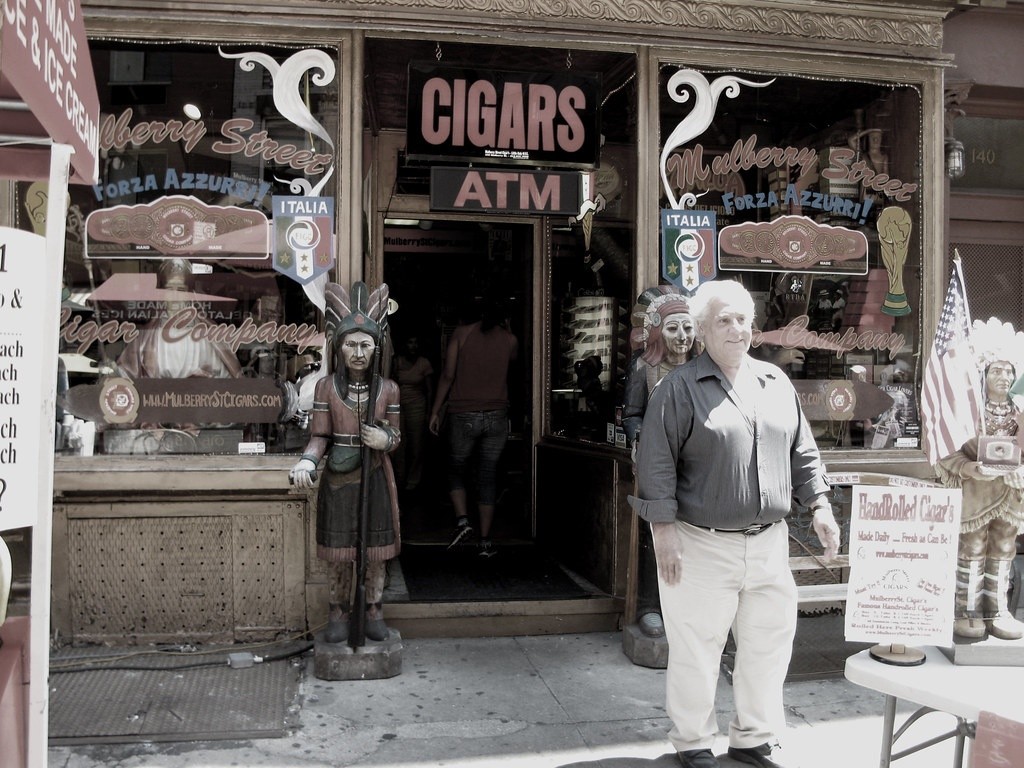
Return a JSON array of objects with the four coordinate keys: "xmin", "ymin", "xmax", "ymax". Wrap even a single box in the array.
[{"xmin": 811, "ymin": 502, "xmax": 833, "ymax": 515}]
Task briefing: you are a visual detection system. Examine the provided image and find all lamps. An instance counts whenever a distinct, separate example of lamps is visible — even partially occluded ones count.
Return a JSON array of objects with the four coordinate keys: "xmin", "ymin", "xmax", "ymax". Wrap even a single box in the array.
[
  {"xmin": 945, "ymin": 137, "xmax": 965, "ymax": 179},
  {"xmin": 183, "ymin": 90, "xmax": 210, "ymax": 121}
]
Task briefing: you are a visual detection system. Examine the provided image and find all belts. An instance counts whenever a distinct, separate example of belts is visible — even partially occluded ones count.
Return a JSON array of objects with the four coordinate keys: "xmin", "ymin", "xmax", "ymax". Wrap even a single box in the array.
[{"xmin": 694, "ymin": 519, "xmax": 783, "ymax": 535}]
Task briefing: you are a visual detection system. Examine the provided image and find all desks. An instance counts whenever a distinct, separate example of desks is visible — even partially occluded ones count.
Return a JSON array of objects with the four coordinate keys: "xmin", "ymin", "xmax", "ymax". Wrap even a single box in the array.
[{"xmin": 844, "ymin": 642, "xmax": 1024, "ymax": 768}]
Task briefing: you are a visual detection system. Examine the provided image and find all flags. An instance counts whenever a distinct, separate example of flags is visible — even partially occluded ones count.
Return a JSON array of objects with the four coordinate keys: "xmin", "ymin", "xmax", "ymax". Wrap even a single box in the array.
[{"xmin": 919, "ymin": 257, "xmax": 983, "ymax": 467}]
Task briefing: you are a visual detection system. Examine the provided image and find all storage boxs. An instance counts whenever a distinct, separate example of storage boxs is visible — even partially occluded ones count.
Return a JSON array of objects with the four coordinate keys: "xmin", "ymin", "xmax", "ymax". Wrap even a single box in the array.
[{"xmin": 768, "ymin": 169, "xmax": 899, "ymax": 387}]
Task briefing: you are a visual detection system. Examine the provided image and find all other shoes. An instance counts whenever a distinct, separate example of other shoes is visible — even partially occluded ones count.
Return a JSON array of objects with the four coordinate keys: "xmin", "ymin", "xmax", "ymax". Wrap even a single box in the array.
[
  {"xmin": 479, "ymin": 539, "xmax": 497, "ymax": 558},
  {"xmin": 446, "ymin": 521, "xmax": 473, "ymax": 550},
  {"xmin": 407, "ymin": 479, "xmax": 416, "ymax": 490},
  {"xmin": 728, "ymin": 741, "xmax": 801, "ymax": 768},
  {"xmin": 678, "ymin": 749, "xmax": 720, "ymax": 768}
]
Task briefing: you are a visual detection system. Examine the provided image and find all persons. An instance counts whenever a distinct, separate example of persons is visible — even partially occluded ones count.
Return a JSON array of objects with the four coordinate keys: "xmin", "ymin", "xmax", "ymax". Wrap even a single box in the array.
[
  {"xmin": 626, "ymin": 284, "xmax": 706, "ymax": 639},
  {"xmin": 391, "ymin": 286, "xmax": 602, "ymax": 560},
  {"xmin": 935, "ymin": 316, "xmax": 1024, "ymax": 640},
  {"xmin": 817, "ymin": 288, "xmax": 846, "ymax": 309},
  {"xmin": 849, "ymin": 126, "xmax": 890, "ymax": 206},
  {"xmin": 287, "ymin": 277, "xmax": 402, "ymax": 644},
  {"xmin": 102, "ymin": 256, "xmax": 252, "ymax": 432},
  {"xmin": 626, "ymin": 279, "xmax": 841, "ymax": 767}
]
